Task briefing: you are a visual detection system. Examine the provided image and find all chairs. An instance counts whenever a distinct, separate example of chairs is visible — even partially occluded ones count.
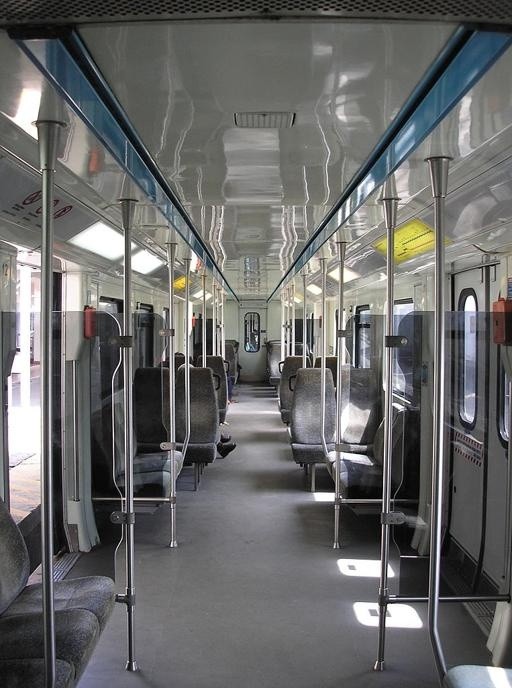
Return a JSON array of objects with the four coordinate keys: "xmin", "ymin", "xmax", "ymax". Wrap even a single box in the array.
[
  {"xmin": 91, "ymin": 340, "xmax": 237, "ymax": 516},
  {"xmin": 263, "ymin": 338, "xmax": 421, "ymax": 520},
  {"xmin": 0, "ymin": 495, "xmax": 116, "ymax": 687}
]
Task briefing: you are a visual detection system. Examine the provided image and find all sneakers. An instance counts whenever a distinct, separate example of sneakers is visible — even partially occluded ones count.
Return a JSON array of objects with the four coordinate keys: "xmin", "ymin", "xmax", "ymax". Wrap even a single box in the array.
[
  {"xmin": 220, "ymin": 433, "xmax": 232, "ymax": 443},
  {"xmin": 217, "ymin": 442, "xmax": 236, "ymax": 458}
]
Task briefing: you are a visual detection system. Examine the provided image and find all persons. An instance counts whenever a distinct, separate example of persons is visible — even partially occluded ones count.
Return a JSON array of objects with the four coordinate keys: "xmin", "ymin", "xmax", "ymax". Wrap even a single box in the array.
[{"xmin": 178, "ymin": 363, "xmax": 237, "ymax": 458}]
